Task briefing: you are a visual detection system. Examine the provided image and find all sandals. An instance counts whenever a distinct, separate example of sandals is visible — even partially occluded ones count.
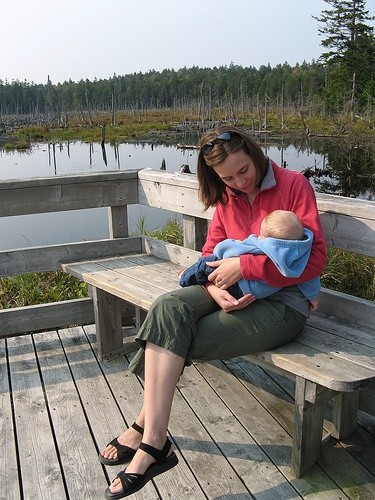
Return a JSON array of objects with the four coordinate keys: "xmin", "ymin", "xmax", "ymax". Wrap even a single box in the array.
[
  {"xmin": 98, "ymin": 421, "xmax": 144, "ymax": 466},
  {"xmin": 104, "ymin": 437, "xmax": 179, "ymax": 500}
]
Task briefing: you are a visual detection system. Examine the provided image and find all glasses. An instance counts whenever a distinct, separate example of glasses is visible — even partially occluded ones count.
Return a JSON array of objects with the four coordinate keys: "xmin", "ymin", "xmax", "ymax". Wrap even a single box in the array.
[{"xmin": 200, "ymin": 131, "xmax": 242, "ymax": 156}]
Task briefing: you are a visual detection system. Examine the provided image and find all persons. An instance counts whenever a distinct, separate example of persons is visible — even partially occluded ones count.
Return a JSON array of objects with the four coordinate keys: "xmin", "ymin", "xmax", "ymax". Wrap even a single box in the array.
[
  {"xmin": 176, "ymin": 209, "xmax": 320, "ymax": 311},
  {"xmin": 98, "ymin": 123, "xmax": 328, "ymax": 500}
]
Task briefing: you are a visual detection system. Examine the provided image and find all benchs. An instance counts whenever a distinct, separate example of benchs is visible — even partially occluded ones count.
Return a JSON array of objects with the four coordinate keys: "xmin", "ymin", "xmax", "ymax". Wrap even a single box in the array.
[{"xmin": 58, "ymin": 168, "xmax": 375, "ymax": 480}]
